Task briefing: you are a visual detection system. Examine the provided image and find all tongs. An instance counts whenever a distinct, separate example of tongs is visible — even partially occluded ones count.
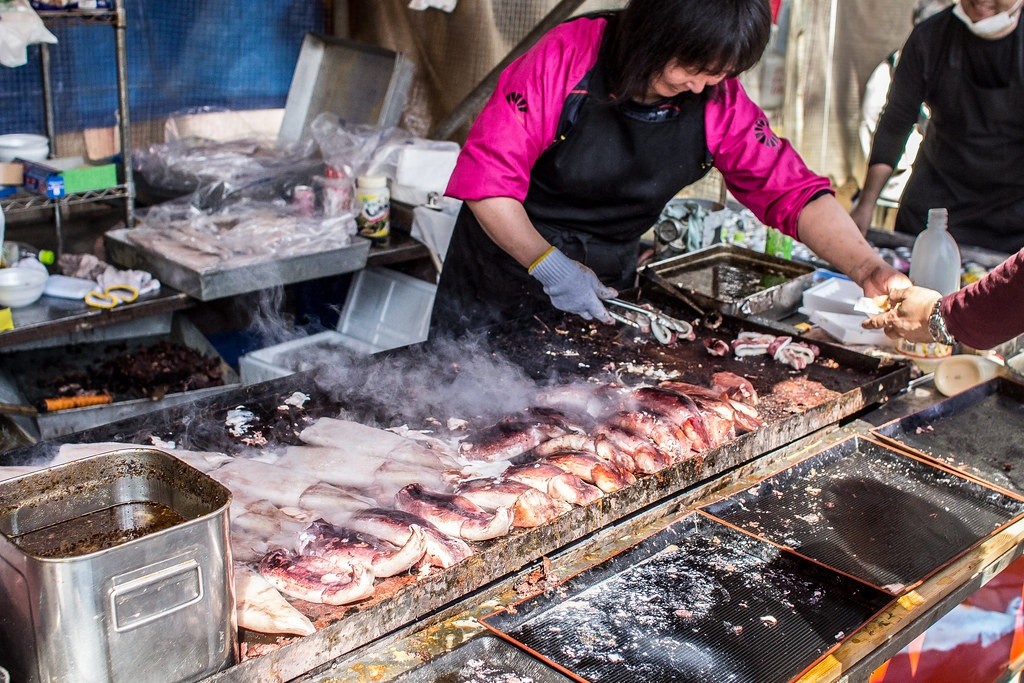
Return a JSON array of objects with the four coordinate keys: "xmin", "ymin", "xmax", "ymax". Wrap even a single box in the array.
[{"xmin": 603, "ymin": 296, "xmax": 688, "ymax": 343}]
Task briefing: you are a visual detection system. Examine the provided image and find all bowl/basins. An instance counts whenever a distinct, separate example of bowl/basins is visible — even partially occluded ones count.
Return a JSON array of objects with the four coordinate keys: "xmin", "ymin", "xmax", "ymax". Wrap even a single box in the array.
[
  {"xmin": 0, "ymin": 267, "xmax": 48, "ymax": 309},
  {"xmin": 0, "ymin": 134, "xmax": 50, "ymax": 162}
]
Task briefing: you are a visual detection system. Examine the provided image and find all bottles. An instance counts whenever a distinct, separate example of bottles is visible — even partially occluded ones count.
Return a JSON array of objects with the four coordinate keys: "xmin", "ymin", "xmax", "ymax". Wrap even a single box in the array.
[
  {"xmin": 1, "ymin": 241, "xmax": 56, "ymax": 267},
  {"xmin": 766, "ymin": 225, "xmax": 794, "ymax": 260},
  {"xmin": 891, "ymin": 207, "xmax": 962, "ymax": 370},
  {"xmin": 354, "ymin": 176, "xmax": 390, "ymax": 239},
  {"xmin": 936, "ymin": 354, "xmax": 1001, "ymax": 398}
]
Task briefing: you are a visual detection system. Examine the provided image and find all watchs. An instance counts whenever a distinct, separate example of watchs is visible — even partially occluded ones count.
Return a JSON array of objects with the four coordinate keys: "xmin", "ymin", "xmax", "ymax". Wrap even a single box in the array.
[{"xmin": 928, "ymin": 298, "xmax": 961, "ymax": 346}]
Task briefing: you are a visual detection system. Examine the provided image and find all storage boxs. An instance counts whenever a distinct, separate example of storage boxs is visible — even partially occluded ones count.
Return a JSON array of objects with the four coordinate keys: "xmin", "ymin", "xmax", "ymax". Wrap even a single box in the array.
[
  {"xmin": 14, "ymin": 158, "xmax": 66, "ymax": 200},
  {"xmin": 802, "ymin": 276, "xmax": 867, "ymax": 317},
  {"xmin": 0, "ymin": 448, "xmax": 238, "ymax": 683},
  {"xmin": 43, "ymin": 155, "xmax": 117, "ymax": 193},
  {"xmin": 809, "ymin": 310, "xmax": 885, "ymax": 347},
  {"xmin": 633, "ymin": 240, "xmax": 818, "ymax": 324},
  {"xmin": 0, "ymin": 313, "xmax": 237, "ymax": 446},
  {"xmin": 238, "ymin": 266, "xmax": 438, "ymax": 387},
  {"xmin": 0, "ymin": 161, "xmax": 25, "ymax": 200},
  {"xmin": 273, "ymin": 27, "xmax": 416, "ymax": 145},
  {"xmin": 102, "ymin": 210, "xmax": 374, "ymax": 305}
]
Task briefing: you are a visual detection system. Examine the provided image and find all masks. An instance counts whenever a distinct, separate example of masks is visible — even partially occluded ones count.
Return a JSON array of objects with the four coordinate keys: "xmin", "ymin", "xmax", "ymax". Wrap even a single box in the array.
[{"xmin": 951, "ymin": 0, "xmax": 1024, "ymax": 42}]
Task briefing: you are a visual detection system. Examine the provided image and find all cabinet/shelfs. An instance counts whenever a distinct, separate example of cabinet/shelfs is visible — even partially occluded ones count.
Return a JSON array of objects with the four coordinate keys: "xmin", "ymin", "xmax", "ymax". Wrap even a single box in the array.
[{"xmin": 0, "ymin": 0, "xmax": 137, "ymax": 264}]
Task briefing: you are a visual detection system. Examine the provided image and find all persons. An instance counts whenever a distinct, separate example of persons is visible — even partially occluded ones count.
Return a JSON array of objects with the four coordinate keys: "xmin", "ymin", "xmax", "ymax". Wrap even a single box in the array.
[
  {"xmin": 858, "ymin": 0, "xmax": 953, "ymax": 232},
  {"xmin": 428, "ymin": 0, "xmax": 912, "ymax": 340},
  {"xmin": 850, "ymin": 0, "xmax": 1024, "ymax": 253},
  {"xmin": 861, "ymin": 246, "xmax": 1024, "ymax": 351}
]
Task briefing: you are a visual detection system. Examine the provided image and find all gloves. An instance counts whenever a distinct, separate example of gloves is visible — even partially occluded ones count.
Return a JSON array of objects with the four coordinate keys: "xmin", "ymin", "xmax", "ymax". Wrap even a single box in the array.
[{"xmin": 527, "ymin": 244, "xmax": 618, "ymax": 327}]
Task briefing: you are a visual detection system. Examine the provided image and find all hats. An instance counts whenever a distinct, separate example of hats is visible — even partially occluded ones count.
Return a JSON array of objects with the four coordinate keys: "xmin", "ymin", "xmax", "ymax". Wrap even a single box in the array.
[{"xmin": 909, "ymin": 0, "xmax": 954, "ymax": 27}]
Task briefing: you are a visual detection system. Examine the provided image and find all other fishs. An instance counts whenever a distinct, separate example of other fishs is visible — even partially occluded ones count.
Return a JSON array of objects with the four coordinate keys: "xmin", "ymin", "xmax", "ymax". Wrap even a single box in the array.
[{"xmin": 1, "ymin": 373, "xmax": 763, "ymax": 637}]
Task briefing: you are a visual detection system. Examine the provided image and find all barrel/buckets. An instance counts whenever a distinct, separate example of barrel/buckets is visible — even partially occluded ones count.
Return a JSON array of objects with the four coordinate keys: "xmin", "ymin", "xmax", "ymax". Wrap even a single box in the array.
[{"xmin": 653, "ymin": 196, "xmax": 726, "ymax": 262}]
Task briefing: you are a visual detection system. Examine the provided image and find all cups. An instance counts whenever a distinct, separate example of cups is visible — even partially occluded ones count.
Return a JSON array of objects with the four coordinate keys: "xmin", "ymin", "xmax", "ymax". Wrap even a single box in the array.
[{"xmin": 741, "ymin": 207, "xmax": 767, "ymax": 252}]
